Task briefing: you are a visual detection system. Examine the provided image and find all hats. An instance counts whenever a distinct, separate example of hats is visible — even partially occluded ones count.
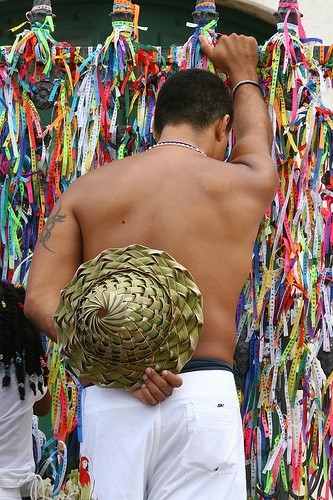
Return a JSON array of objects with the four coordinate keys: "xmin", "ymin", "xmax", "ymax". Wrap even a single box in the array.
[{"xmin": 49, "ymin": 242, "xmax": 206, "ymax": 396}]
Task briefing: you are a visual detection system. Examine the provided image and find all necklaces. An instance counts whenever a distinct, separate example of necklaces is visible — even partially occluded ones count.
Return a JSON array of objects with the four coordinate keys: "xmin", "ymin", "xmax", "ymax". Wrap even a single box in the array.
[{"xmin": 145, "ymin": 140, "xmax": 210, "ymax": 163}]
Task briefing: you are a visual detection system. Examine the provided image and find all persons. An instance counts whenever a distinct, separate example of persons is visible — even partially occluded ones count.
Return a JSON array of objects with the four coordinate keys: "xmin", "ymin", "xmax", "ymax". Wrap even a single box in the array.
[
  {"xmin": 1, "ymin": 280, "xmax": 51, "ymax": 498},
  {"xmin": 21, "ymin": 35, "xmax": 290, "ymax": 500}
]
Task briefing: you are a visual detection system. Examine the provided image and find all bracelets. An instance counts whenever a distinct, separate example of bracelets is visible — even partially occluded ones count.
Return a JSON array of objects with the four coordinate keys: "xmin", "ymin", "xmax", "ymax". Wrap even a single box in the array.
[{"xmin": 230, "ymin": 78, "xmax": 267, "ymax": 102}]
